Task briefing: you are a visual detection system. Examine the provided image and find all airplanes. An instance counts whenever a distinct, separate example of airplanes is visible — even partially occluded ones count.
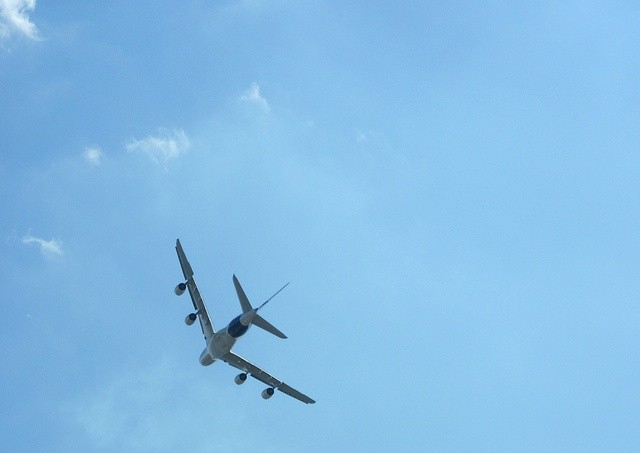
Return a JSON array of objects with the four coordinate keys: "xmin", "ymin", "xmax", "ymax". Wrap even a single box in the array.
[{"xmin": 173, "ymin": 238, "xmax": 316, "ymax": 405}]
